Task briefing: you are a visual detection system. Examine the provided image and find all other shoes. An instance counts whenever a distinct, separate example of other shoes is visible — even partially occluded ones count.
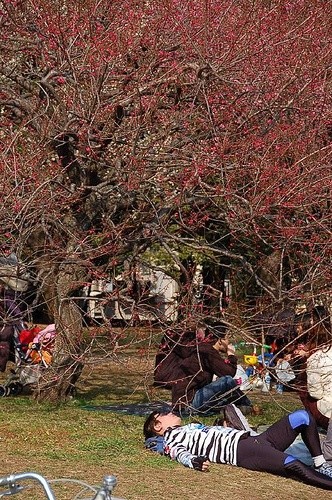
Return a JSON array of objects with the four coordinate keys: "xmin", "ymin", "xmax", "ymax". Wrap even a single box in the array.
[{"xmin": 313, "ymin": 461, "xmax": 332, "ymax": 478}]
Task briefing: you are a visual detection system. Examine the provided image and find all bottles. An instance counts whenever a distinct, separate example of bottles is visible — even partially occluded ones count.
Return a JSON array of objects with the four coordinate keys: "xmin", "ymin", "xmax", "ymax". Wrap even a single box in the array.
[
  {"xmin": 257, "ymin": 355, "xmax": 263, "ymax": 376},
  {"xmin": 265, "ymin": 372, "xmax": 271, "ymax": 390},
  {"xmin": 248, "ymin": 372, "xmax": 254, "ymax": 385},
  {"xmin": 276, "ymin": 379, "xmax": 283, "ymax": 394}
]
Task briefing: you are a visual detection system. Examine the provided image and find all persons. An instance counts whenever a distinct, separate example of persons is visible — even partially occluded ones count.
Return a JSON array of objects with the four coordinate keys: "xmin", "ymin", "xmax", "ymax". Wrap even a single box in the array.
[
  {"xmin": 265, "ymin": 307, "xmax": 328, "ymax": 390},
  {"xmin": 0, "ymin": 280, "xmax": 28, "ymax": 397},
  {"xmin": 144, "ymin": 408, "xmax": 332, "ymax": 491},
  {"xmin": 172, "ymin": 322, "xmax": 263, "ymax": 416},
  {"xmin": 297, "ymin": 322, "xmax": 332, "ymax": 430},
  {"xmin": 152, "ymin": 318, "xmax": 214, "ymax": 391}
]
